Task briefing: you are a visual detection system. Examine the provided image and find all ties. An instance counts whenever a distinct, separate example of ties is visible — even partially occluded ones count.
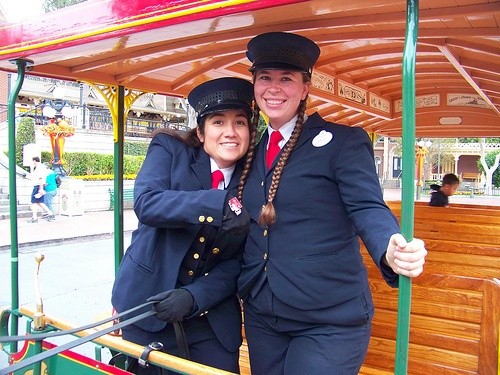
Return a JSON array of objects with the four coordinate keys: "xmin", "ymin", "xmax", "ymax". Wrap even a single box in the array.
[
  {"xmin": 211, "ymin": 169, "xmax": 224, "ymax": 188},
  {"xmin": 266, "ymin": 130, "xmax": 283, "ymax": 170}
]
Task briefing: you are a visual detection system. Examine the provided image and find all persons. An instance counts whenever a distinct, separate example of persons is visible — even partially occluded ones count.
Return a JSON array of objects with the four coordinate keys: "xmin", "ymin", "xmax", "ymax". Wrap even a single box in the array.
[
  {"xmin": 41, "ymin": 162, "xmax": 59, "ymax": 222},
  {"xmin": 111, "ymin": 77, "xmax": 254, "ymax": 375},
  {"xmin": 429, "ymin": 173, "xmax": 460, "ymax": 207},
  {"xmin": 237, "ymin": 31, "xmax": 428, "ymax": 375},
  {"xmin": 22, "ymin": 157, "xmax": 55, "ymax": 223}
]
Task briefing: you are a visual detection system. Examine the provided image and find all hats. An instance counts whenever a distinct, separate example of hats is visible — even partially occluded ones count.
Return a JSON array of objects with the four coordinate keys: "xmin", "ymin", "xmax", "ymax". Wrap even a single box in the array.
[
  {"xmin": 245, "ymin": 30, "xmax": 321, "ymax": 71},
  {"xmin": 188, "ymin": 77, "xmax": 255, "ymax": 117}
]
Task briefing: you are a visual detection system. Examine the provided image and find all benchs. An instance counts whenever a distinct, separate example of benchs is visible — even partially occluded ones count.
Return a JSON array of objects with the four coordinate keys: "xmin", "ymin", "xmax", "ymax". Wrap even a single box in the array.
[
  {"xmin": 108, "ymin": 188, "xmax": 135, "ymax": 211},
  {"xmin": 238, "ymin": 200, "xmax": 500, "ymax": 375},
  {"xmin": 124, "ymin": 125, "xmax": 151, "ymax": 137},
  {"xmin": 462, "ymin": 173, "xmax": 482, "ymax": 182}
]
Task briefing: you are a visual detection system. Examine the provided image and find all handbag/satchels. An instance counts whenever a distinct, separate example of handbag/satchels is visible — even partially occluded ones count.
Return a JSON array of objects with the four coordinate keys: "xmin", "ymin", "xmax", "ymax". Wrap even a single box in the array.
[{"xmin": 56, "ymin": 174, "xmax": 61, "ymax": 186}]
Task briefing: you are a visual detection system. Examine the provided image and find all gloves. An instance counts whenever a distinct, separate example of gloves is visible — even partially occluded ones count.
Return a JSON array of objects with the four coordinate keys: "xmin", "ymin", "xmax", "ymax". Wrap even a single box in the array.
[
  {"xmin": 222, "ymin": 192, "xmax": 250, "ymax": 235},
  {"xmin": 147, "ymin": 288, "xmax": 194, "ymax": 323}
]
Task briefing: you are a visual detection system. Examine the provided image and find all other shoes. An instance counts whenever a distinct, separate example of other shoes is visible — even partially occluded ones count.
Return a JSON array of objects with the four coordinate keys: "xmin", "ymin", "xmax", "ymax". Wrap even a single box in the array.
[
  {"xmin": 28, "ymin": 217, "xmax": 38, "ymax": 223},
  {"xmin": 43, "ymin": 213, "xmax": 56, "ymax": 219}
]
